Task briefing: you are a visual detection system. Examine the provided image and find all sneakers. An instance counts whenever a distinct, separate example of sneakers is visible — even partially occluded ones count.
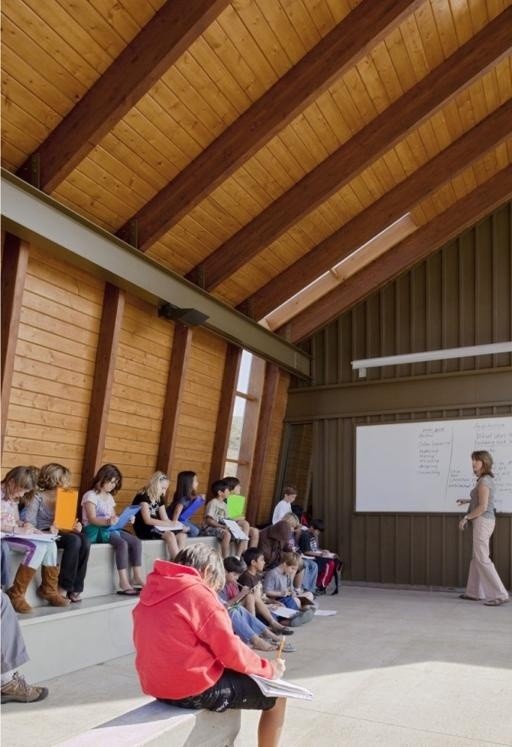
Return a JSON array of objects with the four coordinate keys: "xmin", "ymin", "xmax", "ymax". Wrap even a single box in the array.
[
  {"xmin": 289, "ymin": 609, "xmax": 314, "ymax": 627},
  {"xmin": 1, "ymin": 672, "xmax": 48, "ymax": 703}
]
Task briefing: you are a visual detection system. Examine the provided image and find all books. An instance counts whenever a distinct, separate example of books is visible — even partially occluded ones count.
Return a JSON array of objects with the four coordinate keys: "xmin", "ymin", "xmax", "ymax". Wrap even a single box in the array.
[
  {"xmin": 246, "ymin": 671, "xmax": 317, "ymax": 704},
  {"xmin": 222, "ymin": 518, "xmax": 250, "ymax": 541},
  {"xmin": 154, "ymin": 518, "xmax": 186, "ymax": 532},
  {"xmin": 10, "ymin": 533, "xmax": 61, "ymax": 544}
]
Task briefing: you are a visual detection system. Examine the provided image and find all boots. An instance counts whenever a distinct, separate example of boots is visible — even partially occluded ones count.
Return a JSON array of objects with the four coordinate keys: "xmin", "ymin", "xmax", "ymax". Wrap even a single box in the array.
[
  {"xmin": 37, "ymin": 566, "xmax": 70, "ymax": 607},
  {"xmin": 6, "ymin": 563, "xmax": 36, "ymax": 614}
]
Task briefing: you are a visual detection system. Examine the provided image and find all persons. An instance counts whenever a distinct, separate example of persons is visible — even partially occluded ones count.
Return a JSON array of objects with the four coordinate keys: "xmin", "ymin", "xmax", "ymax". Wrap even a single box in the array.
[
  {"xmin": 199, "ymin": 475, "xmax": 344, "ymax": 652},
  {"xmin": 80, "ymin": 462, "xmax": 146, "ymax": 596},
  {"xmin": 2, "ymin": 589, "xmax": 49, "ymax": 703},
  {"xmin": 130, "ymin": 470, "xmax": 191, "ymax": 562},
  {"xmin": 130, "ymin": 541, "xmax": 289, "ymax": 746},
  {"xmin": 270, "ymin": 485, "xmax": 297, "ymax": 525},
  {"xmin": 23, "ymin": 460, "xmax": 91, "ymax": 603},
  {"xmin": 2, "ymin": 465, "xmax": 69, "ymax": 611},
  {"xmin": 165, "ymin": 471, "xmax": 206, "ymax": 537},
  {"xmin": 455, "ymin": 448, "xmax": 511, "ymax": 607}
]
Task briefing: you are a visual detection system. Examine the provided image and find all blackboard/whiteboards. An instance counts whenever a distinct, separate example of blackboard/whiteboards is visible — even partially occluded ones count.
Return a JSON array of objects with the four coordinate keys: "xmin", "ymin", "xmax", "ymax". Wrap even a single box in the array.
[{"xmin": 352, "ymin": 413, "xmax": 512, "ymax": 514}]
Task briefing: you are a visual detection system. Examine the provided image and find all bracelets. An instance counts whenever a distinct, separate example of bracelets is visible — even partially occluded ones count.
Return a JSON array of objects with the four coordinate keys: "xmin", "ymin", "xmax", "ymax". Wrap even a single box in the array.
[{"xmin": 463, "ymin": 515, "xmax": 470, "ymax": 522}]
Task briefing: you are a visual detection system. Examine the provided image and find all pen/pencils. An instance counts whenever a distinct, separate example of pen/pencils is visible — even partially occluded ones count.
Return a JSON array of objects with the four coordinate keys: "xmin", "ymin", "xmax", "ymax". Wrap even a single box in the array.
[{"xmin": 276, "ymin": 635, "xmax": 286, "ymax": 656}]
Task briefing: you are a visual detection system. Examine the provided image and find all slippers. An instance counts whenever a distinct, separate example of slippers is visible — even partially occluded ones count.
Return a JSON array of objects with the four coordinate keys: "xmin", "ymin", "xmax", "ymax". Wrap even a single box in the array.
[
  {"xmin": 117, "ymin": 589, "xmax": 139, "ymax": 595},
  {"xmin": 270, "ymin": 626, "xmax": 293, "ymax": 635},
  {"xmin": 267, "ymin": 638, "xmax": 296, "ymax": 652},
  {"xmin": 483, "ymin": 598, "xmax": 510, "ymax": 606},
  {"xmin": 69, "ymin": 592, "xmax": 82, "ymax": 602}
]
